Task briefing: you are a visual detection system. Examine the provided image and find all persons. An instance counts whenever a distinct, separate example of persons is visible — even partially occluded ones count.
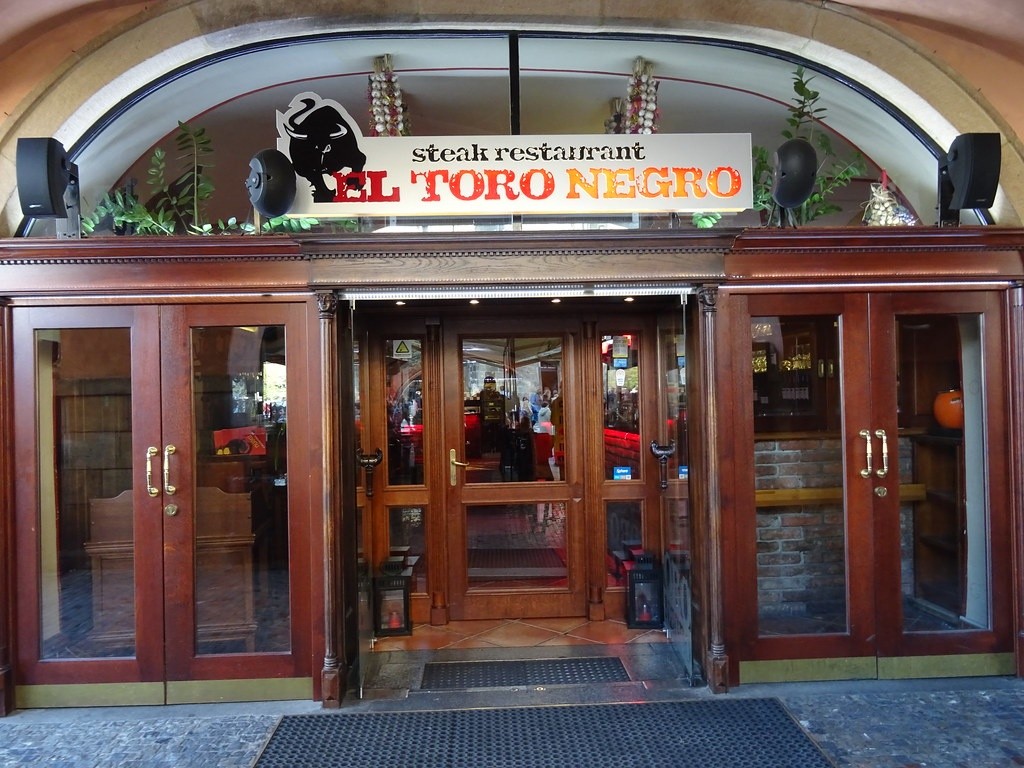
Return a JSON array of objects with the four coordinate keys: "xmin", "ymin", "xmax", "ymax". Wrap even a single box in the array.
[
  {"xmin": 518, "ymin": 386, "xmax": 558, "ymax": 434},
  {"xmin": 388, "ymin": 390, "xmax": 423, "ymax": 456},
  {"xmin": 604, "ymin": 388, "xmax": 632, "ymax": 422},
  {"xmin": 517, "ymin": 417, "xmax": 537, "ymax": 481},
  {"xmin": 472, "ymin": 376, "xmax": 521, "ymax": 462},
  {"xmin": 500, "ymin": 385, "xmax": 520, "ymax": 426}
]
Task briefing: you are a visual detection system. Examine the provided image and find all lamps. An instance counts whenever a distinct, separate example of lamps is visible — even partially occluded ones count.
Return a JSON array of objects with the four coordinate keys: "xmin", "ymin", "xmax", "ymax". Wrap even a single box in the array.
[
  {"xmin": 14, "ymin": 137, "xmax": 82, "ymax": 240},
  {"xmin": 937, "ymin": 132, "xmax": 1002, "ymax": 227},
  {"xmin": 766, "ymin": 140, "xmax": 818, "ymax": 228},
  {"xmin": 244, "ymin": 147, "xmax": 296, "ymax": 235}
]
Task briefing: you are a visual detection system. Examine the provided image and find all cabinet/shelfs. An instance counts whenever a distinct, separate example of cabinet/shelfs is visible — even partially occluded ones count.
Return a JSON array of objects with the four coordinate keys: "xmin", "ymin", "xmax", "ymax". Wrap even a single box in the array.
[{"xmin": 908, "ymin": 434, "xmax": 968, "ymax": 617}]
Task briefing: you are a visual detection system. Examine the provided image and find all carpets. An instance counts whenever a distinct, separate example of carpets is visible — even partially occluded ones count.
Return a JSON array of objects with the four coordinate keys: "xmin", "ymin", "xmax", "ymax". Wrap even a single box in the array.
[{"xmin": 250, "ymin": 698, "xmax": 837, "ymax": 768}]
